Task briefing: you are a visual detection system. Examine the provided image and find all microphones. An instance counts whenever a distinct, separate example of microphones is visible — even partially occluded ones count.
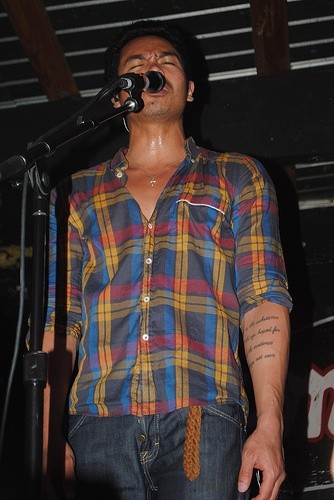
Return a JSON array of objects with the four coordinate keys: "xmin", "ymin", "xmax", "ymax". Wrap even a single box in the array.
[{"xmin": 119, "ymin": 70, "xmax": 166, "ymax": 94}]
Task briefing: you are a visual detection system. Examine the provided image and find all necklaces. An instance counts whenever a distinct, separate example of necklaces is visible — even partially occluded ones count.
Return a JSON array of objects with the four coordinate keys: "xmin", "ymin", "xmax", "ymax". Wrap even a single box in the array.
[{"xmin": 131, "ymin": 156, "xmax": 178, "ymax": 188}]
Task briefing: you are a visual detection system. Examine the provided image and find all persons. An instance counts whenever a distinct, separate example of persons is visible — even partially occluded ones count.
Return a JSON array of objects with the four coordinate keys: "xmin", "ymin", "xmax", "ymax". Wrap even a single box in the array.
[{"xmin": 23, "ymin": 19, "xmax": 294, "ymax": 500}]
